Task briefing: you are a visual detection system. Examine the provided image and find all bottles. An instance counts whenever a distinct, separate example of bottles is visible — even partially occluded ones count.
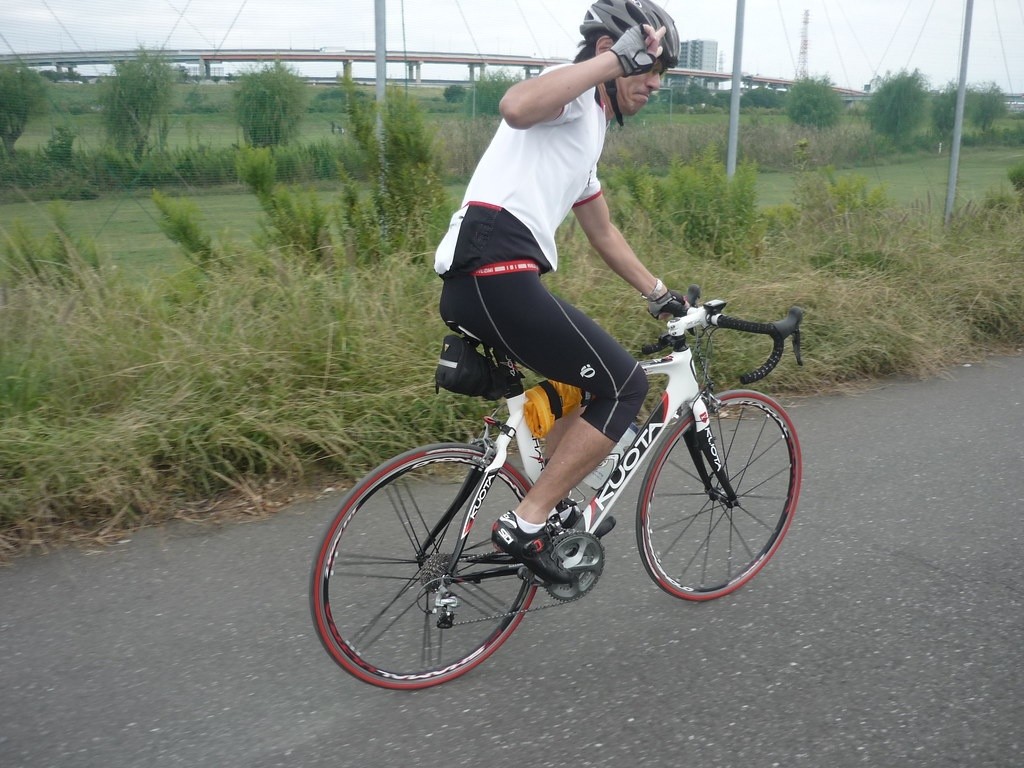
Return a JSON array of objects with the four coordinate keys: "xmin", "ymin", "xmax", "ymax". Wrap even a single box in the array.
[{"xmin": 581, "ymin": 415, "xmax": 644, "ymax": 490}]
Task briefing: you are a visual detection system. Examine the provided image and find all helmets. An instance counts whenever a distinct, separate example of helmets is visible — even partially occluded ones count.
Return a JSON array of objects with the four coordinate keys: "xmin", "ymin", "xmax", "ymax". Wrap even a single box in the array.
[{"xmin": 580, "ymin": 0, "xmax": 681, "ymax": 68}]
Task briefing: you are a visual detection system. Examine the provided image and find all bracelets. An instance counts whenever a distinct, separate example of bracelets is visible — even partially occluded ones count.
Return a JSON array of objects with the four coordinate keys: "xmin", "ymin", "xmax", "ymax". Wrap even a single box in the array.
[{"xmin": 640, "ymin": 278, "xmax": 662, "ymax": 300}]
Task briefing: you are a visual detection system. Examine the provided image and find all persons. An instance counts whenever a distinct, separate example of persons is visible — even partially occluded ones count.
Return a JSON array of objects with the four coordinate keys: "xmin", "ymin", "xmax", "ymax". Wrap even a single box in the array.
[
  {"xmin": 433, "ymin": 0, "xmax": 690, "ymax": 583},
  {"xmin": 331, "ymin": 121, "xmax": 335, "ymax": 134}
]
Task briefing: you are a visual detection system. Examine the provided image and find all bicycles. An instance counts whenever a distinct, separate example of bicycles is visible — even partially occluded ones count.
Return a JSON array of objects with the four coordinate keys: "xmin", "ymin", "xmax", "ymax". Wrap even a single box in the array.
[{"xmin": 306, "ymin": 284, "xmax": 803, "ymax": 691}]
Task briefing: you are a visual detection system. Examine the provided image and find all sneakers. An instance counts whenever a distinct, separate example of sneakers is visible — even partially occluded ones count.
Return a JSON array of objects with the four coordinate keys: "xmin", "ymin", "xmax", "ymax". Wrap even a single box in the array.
[
  {"xmin": 550, "ymin": 504, "xmax": 615, "ymax": 563},
  {"xmin": 491, "ymin": 511, "xmax": 579, "ymax": 583}
]
